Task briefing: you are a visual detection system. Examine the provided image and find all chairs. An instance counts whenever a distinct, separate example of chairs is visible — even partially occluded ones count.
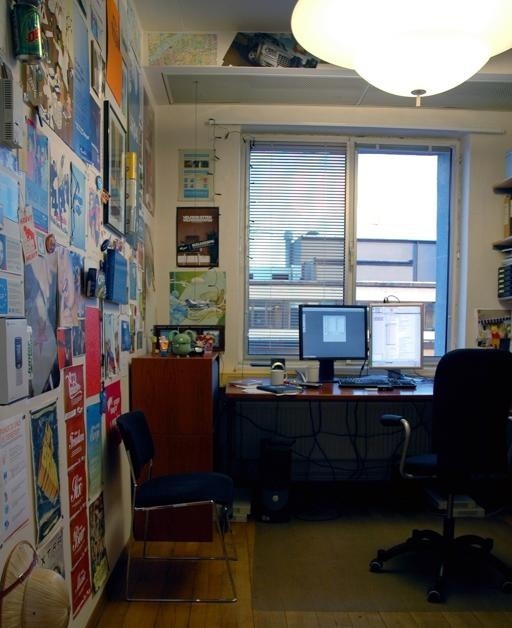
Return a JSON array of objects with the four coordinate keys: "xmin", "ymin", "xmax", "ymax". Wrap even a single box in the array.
[
  {"xmin": 371, "ymin": 350, "xmax": 512, "ymax": 607},
  {"xmin": 114, "ymin": 411, "xmax": 239, "ymax": 605}
]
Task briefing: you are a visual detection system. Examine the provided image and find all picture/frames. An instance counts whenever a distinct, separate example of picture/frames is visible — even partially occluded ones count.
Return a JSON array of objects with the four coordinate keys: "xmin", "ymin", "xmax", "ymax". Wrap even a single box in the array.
[
  {"xmin": 151, "ymin": 324, "xmax": 224, "ymax": 353},
  {"xmin": 101, "ymin": 97, "xmax": 130, "ymax": 240},
  {"xmin": 90, "ymin": 37, "xmax": 107, "ymax": 102},
  {"xmin": 174, "ymin": 204, "xmax": 220, "ymax": 269}
]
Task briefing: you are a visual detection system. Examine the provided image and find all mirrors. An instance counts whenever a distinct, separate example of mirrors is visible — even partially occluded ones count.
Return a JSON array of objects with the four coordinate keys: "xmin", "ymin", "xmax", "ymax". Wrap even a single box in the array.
[{"xmin": 224, "ymin": 376, "xmax": 441, "ymax": 519}]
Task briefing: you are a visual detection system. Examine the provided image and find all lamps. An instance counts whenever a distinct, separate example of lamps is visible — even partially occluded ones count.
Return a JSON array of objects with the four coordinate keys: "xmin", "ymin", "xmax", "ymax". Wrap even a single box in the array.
[{"xmin": 285, "ymin": 1, "xmax": 512, "ymax": 109}]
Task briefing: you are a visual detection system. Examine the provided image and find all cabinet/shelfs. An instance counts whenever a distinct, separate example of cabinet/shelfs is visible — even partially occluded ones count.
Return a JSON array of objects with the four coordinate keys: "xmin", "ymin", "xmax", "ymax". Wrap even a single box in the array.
[
  {"xmin": 131, "ymin": 348, "xmax": 221, "ymax": 547},
  {"xmin": 495, "ymin": 178, "xmax": 512, "ymax": 302}
]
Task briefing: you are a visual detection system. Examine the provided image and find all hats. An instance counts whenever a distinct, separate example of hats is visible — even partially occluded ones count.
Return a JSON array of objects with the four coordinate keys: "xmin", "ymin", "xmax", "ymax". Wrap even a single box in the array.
[{"xmin": 1, "ymin": 539, "xmax": 70, "ymax": 628}]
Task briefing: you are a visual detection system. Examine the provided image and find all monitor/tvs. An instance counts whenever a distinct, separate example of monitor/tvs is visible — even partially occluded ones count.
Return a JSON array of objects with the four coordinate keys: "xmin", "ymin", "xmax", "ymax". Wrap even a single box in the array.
[
  {"xmin": 367, "ymin": 301, "xmax": 426, "ymax": 377},
  {"xmin": 298, "ymin": 304, "xmax": 368, "ymax": 384}
]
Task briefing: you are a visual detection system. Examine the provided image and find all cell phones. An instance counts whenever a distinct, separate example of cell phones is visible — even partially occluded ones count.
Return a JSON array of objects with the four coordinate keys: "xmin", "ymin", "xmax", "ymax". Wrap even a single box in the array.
[{"xmin": 299, "ymin": 381, "xmax": 323, "ymax": 388}]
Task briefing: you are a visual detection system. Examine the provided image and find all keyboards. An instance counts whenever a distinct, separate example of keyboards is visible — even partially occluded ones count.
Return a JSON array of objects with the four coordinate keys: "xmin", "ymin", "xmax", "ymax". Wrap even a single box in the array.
[{"xmin": 336, "ymin": 377, "xmax": 417, "ymax": 389}]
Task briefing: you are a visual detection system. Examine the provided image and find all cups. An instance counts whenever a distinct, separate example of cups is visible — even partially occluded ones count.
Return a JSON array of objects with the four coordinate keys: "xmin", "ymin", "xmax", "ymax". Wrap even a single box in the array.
[{"xmin": 270, "ymin": 370, "xmax": 289, "ymax": 386}]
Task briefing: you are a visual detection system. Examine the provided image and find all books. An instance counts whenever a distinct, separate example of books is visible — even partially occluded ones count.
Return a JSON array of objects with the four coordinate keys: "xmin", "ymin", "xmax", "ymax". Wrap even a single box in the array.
[
  {"xmin": 257, "ymin": 385, "xmax": 302, "ymax": 393},
  {"xmin": 229, "ymin": 380, "xmax": 262, "ymax": 386}
]
